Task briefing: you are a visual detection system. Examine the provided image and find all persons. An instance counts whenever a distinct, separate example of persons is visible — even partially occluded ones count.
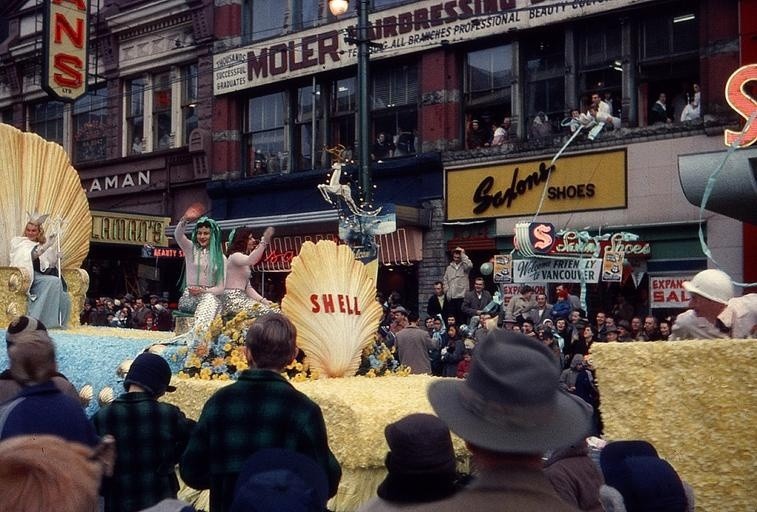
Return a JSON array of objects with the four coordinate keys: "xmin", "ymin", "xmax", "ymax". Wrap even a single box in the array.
[
  {"xmin": 584, "ymin": 438, "xmax": 695, "ymax": 511},
  {"xmin": 1, "ymin": 315, "xmax": 101, "ymax": 446},
  {"xmin": 131, "ymin": 136, "xmax": 143, "ymax": 155},
  {"xmin": 0, "ymin": 435, "xmax": 103, "ymax": 511},
  {"xmin": 377, "ymin": 248, "xmax": 671, "ymax": 380},
  {"xmin": 90, "ymin": 353, "xmax": 196, "ymax": 512},
  {"xmin": 225, "ymin": 225, "xmax": 278, "ymax": 316},
  {"xmin": 392, "ymin": 328, "xmax": 607, "ymax": 512},
  {"xmin": 80, "ymin": 295, "xmax": 171, "ymax": 331},
  {"xmin": 649, "ymin": 91, "xmax": 676, "ymax": 125},
  {"xmin": 8, "ymin": 208, "xmax": 68, "ymax": 327},
  {"xmin": 360, "ymin": 413, "xmax": 466, "ymax": 511},
  {"xmin": 670, "ymin": 269, "xmax": 757, "ymax": 342},
  {"xmin": 680, "ymin": 83, "xmax": 700, "ymax": 121},
  {"xmin": 174, "ymin": 205, "xmax": 227, "ymax": 345},
  {"xmin": 466, "ymin": 89, "xmax": 621, "ymax": 149},
  {"xmin": 179, "ymin": 313, "xmax": 342, "ymax": 511},
  {"xmin": 370, "ymin": 132, "xmax": 396, "ymax": 160}
]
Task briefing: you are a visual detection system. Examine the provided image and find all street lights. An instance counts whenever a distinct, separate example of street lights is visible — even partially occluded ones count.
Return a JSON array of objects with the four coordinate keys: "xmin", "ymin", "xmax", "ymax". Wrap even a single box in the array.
[{"xmin": 328, "ymin": 0, "xmax": 373, "ymax": 207}]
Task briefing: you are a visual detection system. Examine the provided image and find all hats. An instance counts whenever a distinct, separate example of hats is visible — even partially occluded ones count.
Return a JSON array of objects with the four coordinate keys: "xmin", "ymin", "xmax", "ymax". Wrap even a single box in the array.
[
  {"xmin": 124, "ymin": 353, "xmax": 176, "ymax": 400},
  {"xmin": 602, "ymin": 325, "xmax": 620, "ymax": 334},
  {"xmin": 428, "ymin": 329, "xmax": 594, "ymax": 454},
  {"xmin": 26, "ymin": 208, "xmax": 50, "ymax": 224},
  {"xmin": 230, "ymin": 448, "xmax": 329, "ymax": 512},
  {"xmin": 392, "ymin": 306, "xmax": 407, "ymax": 313},
  {"xmin": 385, "ymin": 414, "xmax": 456, "ymax": 487},
  {"xmin": 6, "ymin": 315, "xmax": 46, "ymax": 346},
  {"xmin": 615, "ymin": 320, "xmax": 633, "ymax": 331},
  {"xmin": 600, "ymin": 440, "xmax": 688, "ymax": 512},
  {"xmin": 681, "ymin": 269, "xmax": 734, "ymax": 304}
]
